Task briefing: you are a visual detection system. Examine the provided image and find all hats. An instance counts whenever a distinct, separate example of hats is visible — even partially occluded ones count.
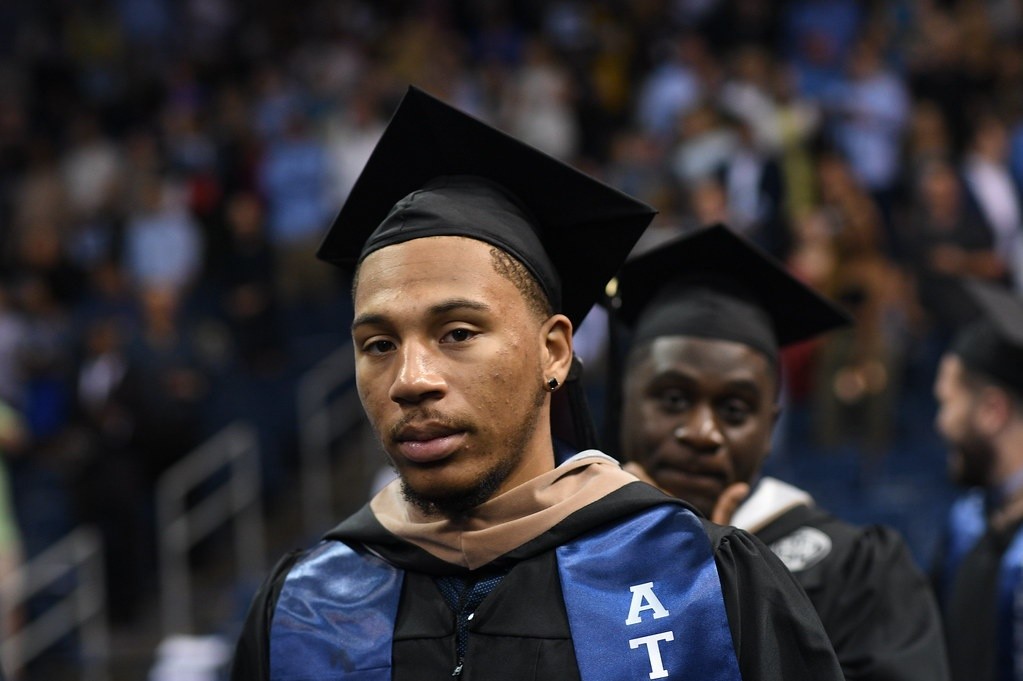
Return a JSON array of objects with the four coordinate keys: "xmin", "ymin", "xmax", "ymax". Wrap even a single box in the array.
[
  {"xmin": 315, "ymin": 83, "xmax": 660, "ymax": 338},
  {"xmin": 595, "ymin": 220, "xmax": 856, "ymax": 375},
  {"xmin": 946, "ymin": 275, "xmax": 1023, "ymax": 389}
]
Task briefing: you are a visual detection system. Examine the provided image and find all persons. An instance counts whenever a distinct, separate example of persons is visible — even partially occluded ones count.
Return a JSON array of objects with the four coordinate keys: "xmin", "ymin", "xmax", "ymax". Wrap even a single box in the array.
[
  {"xmin": 0, "ymin": 0, "xmax": 1023, "ymax": 681},
  {"xmin": 223, "ymin": 86, "xmax": 846, "ymax": 681},
  {"xmin": 609, "ymin": 220, "xmax": 950, "ymax": 681}
]
